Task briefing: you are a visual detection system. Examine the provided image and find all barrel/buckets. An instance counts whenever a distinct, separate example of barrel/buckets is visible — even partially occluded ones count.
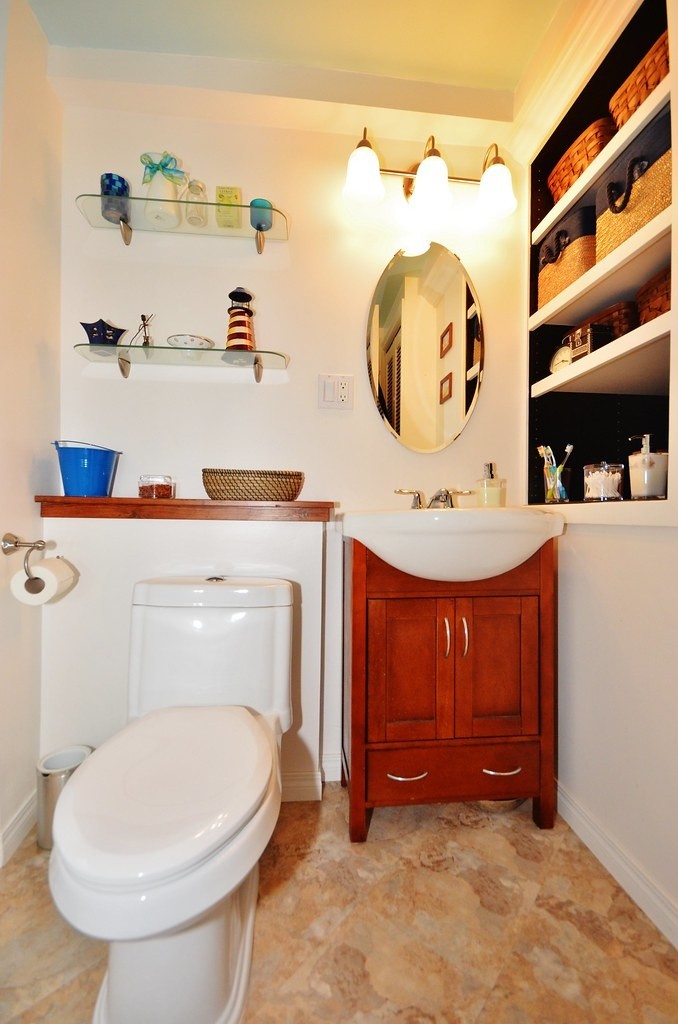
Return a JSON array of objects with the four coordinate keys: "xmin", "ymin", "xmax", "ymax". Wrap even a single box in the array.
[{"xmin": 50, "ymin": 440, "xmax": 124, "ymax": 499}]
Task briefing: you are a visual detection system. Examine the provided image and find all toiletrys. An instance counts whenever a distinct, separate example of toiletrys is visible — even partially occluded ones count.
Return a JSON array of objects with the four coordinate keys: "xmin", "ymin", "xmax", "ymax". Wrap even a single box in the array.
[{"xmin": 625, "ymin": 433, "xmax": 668, "ymax": 500}]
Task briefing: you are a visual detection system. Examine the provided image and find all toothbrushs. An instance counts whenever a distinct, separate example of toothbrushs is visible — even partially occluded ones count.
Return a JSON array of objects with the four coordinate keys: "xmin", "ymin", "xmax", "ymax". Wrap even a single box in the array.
[
  {"xmin": 546, "ymin": 443, "xmax": 573, "ymax": 499},
  {"xmin": 537, "ymin": 445, "xmax": 566, "ymax": 500}
]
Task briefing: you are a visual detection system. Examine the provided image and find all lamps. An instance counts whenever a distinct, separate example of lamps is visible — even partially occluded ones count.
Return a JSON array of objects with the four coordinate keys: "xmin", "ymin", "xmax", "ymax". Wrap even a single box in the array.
[{"xmin": 343, "ymin": 127, "xmax": 518, "ymax": 217}]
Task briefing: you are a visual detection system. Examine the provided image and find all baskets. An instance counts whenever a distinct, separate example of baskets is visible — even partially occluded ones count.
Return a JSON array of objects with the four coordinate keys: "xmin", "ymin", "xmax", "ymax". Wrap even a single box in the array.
[
  {"xmin": 202, "ymin": 468, "xmax": 303, "ymax": 502},
  {"xmin": 605, "ymin": 30, "xmax": 670, "ymax": 122},
  {"xmin": 546, "ymin": 120, "xmax": 615, "ymax": 204}
]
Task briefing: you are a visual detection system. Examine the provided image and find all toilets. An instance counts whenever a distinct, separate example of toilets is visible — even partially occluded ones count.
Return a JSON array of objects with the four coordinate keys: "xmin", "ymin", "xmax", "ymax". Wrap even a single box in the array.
[{"xmin": 45, "ymin": 574, "xmax": 292, "ymax": 1024}]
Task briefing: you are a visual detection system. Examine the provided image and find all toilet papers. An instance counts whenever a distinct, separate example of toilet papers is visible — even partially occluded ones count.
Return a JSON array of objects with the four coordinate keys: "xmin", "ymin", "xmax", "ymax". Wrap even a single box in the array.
[{"xmin": 10, "ymin": 558, "xmax": 78, "ymax": 606}]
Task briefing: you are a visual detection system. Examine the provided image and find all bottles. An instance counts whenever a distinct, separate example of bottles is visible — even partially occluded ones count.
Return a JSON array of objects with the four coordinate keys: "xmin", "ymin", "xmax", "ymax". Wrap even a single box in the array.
[
  {"xmin": 628, "ymin": 433, "xmax": 668, "ymax": 501},
  {"xmin": 185, "ymin": 181, "xmax": 207, "ymax": 227}
]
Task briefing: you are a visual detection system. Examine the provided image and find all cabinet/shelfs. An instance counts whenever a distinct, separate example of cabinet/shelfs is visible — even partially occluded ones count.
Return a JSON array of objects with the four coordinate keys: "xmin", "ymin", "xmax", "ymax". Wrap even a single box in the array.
[
  {"xmin": 340, "ymin": 537, "xmax": 559, "ymax": 844},
  {"xmin": 524, "ymin": 0, "xmax": 678, "ymax": 526},
  {"xmin": 465, "ymin": 283, "xmax": 484, "ymax": 415}
]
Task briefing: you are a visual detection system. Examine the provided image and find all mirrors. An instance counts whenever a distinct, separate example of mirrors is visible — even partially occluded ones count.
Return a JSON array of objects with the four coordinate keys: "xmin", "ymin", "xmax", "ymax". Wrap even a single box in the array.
[
  {"xmin": 439, "ymin": 323, "xmax": 453, "ymax": 358},
  {"xmin": 365, "ymin": 242, "xmax": 486, "ymax": 456},
  {"xmin": 440, "ymin": 372, "xmax": 452, "ymax": 404}
]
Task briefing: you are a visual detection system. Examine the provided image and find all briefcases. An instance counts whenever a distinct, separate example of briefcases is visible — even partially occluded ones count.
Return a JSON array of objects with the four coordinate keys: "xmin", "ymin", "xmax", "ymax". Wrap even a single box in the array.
[
  {"xmin": 595, "ymin": 113, "xmax": 674, "ymax": 263},
  {"xmin": 536, "ymin": 205, "xmax": 595, "ymax": 309}
]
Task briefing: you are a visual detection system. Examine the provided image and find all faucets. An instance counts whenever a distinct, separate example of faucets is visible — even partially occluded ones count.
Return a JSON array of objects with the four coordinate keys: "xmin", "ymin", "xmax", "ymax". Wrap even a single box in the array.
[{"xmin": 427, "ymin": 488, "xmax": 450, "ymax": 509}]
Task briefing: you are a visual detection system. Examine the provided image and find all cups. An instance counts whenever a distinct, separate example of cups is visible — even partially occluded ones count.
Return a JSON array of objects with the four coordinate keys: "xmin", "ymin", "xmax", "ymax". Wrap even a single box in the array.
[
  {"xmin": 138, "ymin": 475, "xmax": 173, "ymax": 500},
  {"xmin": 140, "ymin": 152, "xmax": 189, "ymax": 229},
  {"xmin": 100, "ymin": 173, "xmax": 130, "ymax": 224},
  {"xmin": 251, "ymin": 199, "xmax": 273, "ymax": 231},
  {"xmin": 583, "ymin": 461, "xmax": 624, "ymax": 502},
  {"xmin": 543, "ymin": 467, "xmax": 570, "ymax": 504}
]
